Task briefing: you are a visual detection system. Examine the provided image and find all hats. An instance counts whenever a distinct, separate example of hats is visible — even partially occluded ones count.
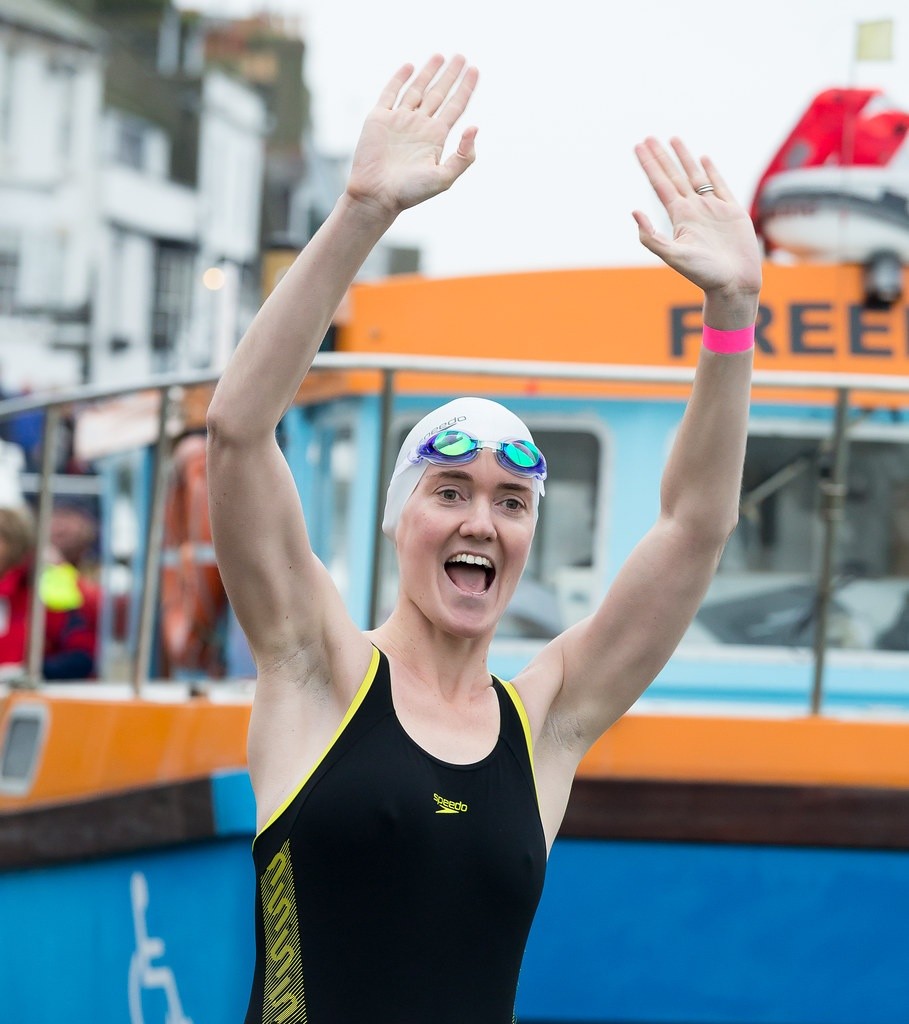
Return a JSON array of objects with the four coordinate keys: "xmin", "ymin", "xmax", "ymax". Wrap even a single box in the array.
[{"xmin": 382, "ymin": 397, "xmax": 579, "ymax": 550}]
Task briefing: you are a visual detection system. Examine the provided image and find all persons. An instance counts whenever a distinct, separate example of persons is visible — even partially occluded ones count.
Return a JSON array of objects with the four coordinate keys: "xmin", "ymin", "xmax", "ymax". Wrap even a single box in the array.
[
  {"xmin": 0, "ymin": 508, "xmax": 102, "ymax": 682},
  {"xmin": 205, "ymin": 53, "xmax": 762, "ymax": 1024}
]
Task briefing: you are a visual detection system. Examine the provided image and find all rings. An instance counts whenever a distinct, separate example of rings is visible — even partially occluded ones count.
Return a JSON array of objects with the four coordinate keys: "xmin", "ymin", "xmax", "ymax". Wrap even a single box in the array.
[{"xmin": 694, "ymin": 184, "xmax": 714, "ymax": 195}]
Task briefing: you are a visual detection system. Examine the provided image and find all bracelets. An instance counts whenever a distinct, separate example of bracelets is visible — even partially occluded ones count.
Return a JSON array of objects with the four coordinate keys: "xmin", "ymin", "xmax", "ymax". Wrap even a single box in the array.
[{"xmin": 702, "ymin": 323, "xmax": 755, "ymax": 355}]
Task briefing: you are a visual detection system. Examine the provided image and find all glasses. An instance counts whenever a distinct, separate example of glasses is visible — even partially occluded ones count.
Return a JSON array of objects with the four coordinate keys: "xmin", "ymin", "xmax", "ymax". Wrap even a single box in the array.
[{"xmin": 409, "ymin": 428, "xmax": 547, "ymax": 477}]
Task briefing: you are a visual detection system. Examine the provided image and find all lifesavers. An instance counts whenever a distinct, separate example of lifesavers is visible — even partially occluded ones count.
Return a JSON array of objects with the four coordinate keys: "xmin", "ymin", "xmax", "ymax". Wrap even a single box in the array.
[{"xmin": 149, "ymin": 431, "xmax": 229, "ymax": 666}]
